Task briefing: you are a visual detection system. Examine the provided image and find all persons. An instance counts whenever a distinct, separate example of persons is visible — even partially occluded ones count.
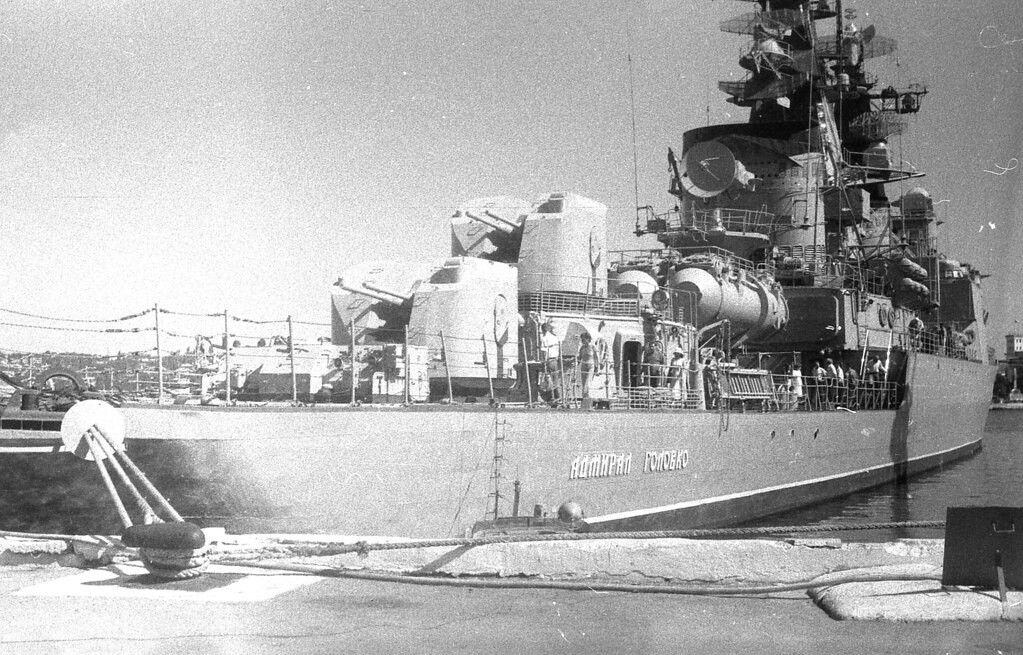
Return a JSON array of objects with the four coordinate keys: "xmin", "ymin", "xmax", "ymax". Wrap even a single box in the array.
[
  {"xmin": 910, "ymin": 313, "xmax": 971, "ymax": 361},
  {"xmin": 639, "ymin": 342, "xmax": 728, "ymax": 408},
  {"xmin": 762, "ymin": 355, "xmax": 887, "ymax": 410},
  {"xmin": 540, "ymin": 322, "xmax": 561, "ymax": 407},
  {"xmin": 674, "ymin": 206, "xmax": 685, "ymax": 228},
  {"xmin": 577, "ymin": 332, "xmax": 601, "ymax": 398}
]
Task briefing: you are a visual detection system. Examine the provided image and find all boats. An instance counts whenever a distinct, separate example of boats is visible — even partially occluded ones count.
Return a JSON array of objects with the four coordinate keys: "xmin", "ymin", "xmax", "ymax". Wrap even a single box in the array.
[{"xmin": 0, "ymin": 0, "xmax": 1001, "ymax": 566}]
